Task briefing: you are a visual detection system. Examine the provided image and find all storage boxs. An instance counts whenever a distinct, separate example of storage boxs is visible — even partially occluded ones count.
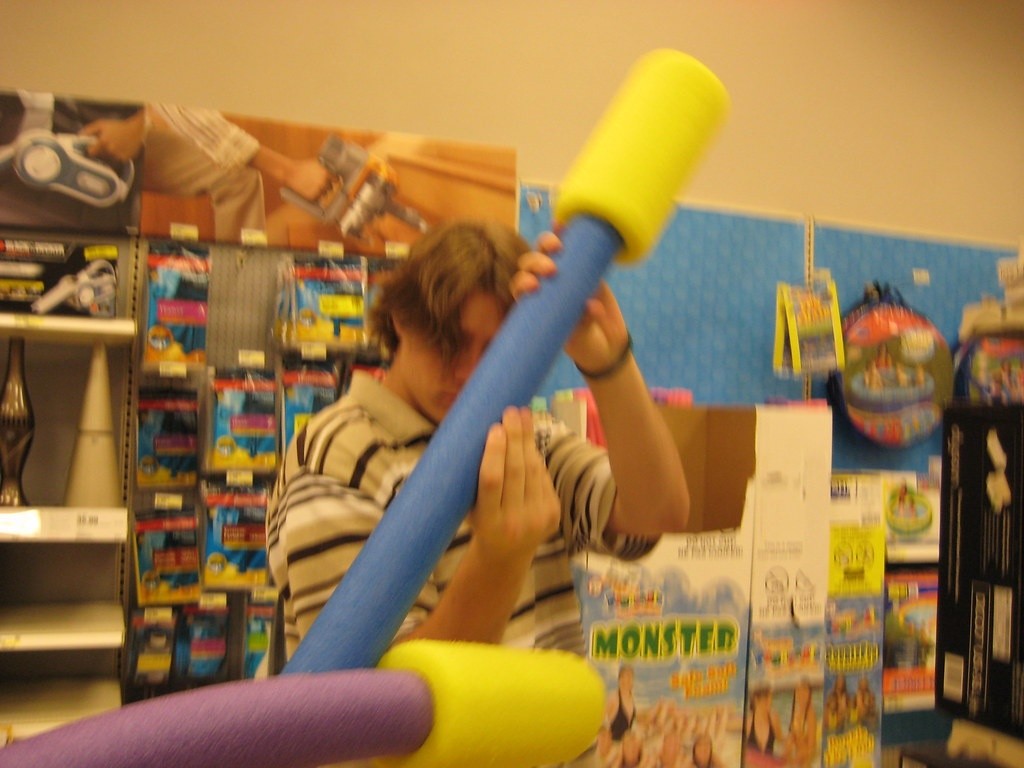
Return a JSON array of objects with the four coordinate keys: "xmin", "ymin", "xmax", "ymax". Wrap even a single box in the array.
[{"xmin": 0, "ymin": 238, "xmax": 119, "ymax": 318}]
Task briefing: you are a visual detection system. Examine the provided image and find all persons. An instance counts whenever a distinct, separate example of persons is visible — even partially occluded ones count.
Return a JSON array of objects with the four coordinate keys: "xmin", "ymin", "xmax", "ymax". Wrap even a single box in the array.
[
  {"xmin": 823, "ymin": 674, "xmax": 877, "ymax": 735},
  {"xmin": 265, "ymin": 214, "xmax": 691, "ymax": 768},
  {"xmin": 142, "ymin": 103, "xmax": 339, "ymax": 246},
  {"xmin": 746, "ymin": 675, "xmax": 818, "ymax": 768},
  {"xmin": 595, "ymin": 663, "xmax": 730, "ymax": 767}
]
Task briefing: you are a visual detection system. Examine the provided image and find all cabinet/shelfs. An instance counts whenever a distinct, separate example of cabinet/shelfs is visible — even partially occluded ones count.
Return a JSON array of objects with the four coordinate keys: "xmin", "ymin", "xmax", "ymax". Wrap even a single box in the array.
[{"xmin": 0, "ymin": 309, "xmax": 140, "ymax": 750}]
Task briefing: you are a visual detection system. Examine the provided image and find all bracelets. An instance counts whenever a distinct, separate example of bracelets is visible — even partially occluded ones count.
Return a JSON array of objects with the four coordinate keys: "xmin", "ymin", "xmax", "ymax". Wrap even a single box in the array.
[{"xmin": 571, "ymin": 324, "xmax": 633, "ymax": 378}]
